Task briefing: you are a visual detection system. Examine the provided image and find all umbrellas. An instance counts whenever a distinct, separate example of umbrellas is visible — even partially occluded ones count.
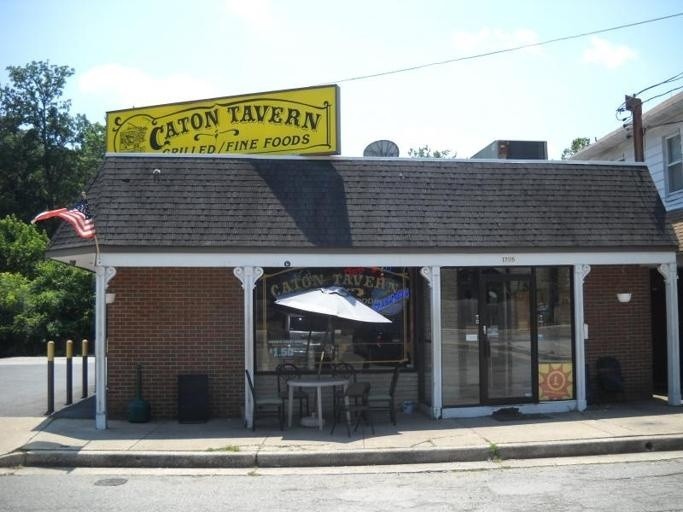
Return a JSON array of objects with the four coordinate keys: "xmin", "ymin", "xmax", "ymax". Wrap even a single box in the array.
[{"xmin": 270, "ymin": 285, "xmax": 395, "ymax": 416}]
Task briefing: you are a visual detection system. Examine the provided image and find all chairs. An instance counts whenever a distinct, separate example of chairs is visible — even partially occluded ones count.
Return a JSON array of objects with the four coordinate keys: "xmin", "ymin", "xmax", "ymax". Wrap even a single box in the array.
[
  {"xmin": 244, "ymin": 362, "xmax": 407, "ymax": 431},
  {"xmin": 596, "ymin": 355, "xmax": 625, "ymax": 404}
]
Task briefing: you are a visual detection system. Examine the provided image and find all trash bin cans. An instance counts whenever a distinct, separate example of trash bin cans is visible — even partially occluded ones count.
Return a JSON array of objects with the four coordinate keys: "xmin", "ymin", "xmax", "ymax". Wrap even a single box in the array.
[{"xmin": 176, "ymin": 374, "xmax": 208, "ymax": 423}]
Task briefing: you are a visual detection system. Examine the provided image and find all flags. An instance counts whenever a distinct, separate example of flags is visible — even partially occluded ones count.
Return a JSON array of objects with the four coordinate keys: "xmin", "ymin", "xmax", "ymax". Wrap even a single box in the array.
[{"xmin": 28, "ymin": 200, "xmax": 95, "ymax": 240}]
